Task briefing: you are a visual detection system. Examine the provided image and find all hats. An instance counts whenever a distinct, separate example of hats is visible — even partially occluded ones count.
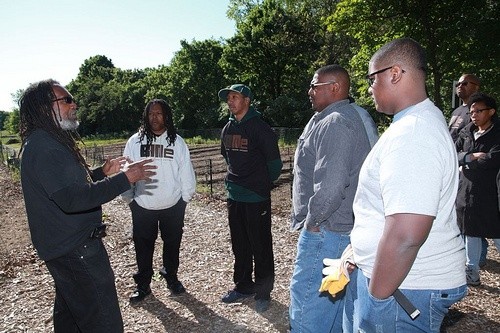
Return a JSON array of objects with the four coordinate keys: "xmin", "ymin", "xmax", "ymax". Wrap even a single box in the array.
[{"xmin": 218, "ymin": 84, "xmax": 252, "ymax": 103}]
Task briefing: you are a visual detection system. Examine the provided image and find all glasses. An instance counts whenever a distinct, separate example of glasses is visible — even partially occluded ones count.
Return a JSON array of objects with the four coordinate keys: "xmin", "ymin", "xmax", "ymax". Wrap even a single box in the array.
[
  {"xmin": 309, "ymin": 82, "xmax": 332, "ymax": 89},
  {"xmin": 49, "ymin": 96, "xmax": 76, "ymax": 105},
  {"xmin": 467, "ymin": 108, "xmax": 490, "ymax": 115},
  {"xmin": 366, "ymin": 66, "xmax": 405, "ymax": 87},
  {"xmin": 455, "ymin": 80, "xmax": 476, "ymax": 88}
]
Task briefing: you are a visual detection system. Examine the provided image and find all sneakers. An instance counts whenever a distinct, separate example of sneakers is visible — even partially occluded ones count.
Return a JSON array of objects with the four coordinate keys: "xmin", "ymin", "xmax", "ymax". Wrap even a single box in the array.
[{"xmin": 465, "ymin": 266, "xmax": 481, "ymax": 286}]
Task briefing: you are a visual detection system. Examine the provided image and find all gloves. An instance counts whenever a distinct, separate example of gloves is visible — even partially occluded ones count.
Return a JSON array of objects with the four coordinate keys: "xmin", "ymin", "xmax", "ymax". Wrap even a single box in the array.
[{"xmin": 318, "ymin": 244, "xmax": 355, "ymax": 298}]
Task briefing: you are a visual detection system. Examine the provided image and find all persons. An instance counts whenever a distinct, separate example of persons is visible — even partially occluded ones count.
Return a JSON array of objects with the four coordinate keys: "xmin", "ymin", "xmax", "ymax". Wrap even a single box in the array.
[
  {"xmin": 218, "ymin": 84, "xmax": 282, "ymax": 313},
  {"xmin": 121, "ymin": 99, "xmax": 195, "ymax": 301},
  {"xmin": 288, "ymin": 65, "xmax": 371, "ymax": 332},
  {"xmin": 18, "ymin": 79, "xmax": 158, "ymax": 333},
  {"xmin": 454, "ymin": 95, "xmax": 500, "ymax": 287},
  {"xmin": 343, "ymin": 37, "xmax": 468, "ymax": 332},
  {"xmin": 447, "ymin": 74, "xmax": 482, "ymax": 146}
]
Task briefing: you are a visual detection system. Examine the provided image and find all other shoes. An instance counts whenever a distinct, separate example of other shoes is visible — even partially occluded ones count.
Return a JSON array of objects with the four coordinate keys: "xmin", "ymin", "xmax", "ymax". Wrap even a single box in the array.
[
  {"xmin": 222, "ymin": 284, "xmax": 257, "ymax": 303},
  {"xmin": 129, "ymin": 286, "xmax": 152, "ymax": 303},
  {"xmin": 167, "ymin": 280, "xmax": 185, "ymax": 294},
  {"xmin": 256, "ymin": 298, "xmax": 269, "ymax": 311}
]
